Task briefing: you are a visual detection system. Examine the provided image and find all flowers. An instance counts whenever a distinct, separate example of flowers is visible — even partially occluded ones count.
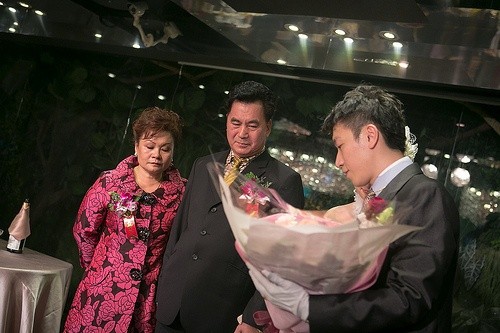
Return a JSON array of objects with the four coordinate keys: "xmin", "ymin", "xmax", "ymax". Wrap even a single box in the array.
[
  {"xmin": 108, "ymin": 191, "xmax": 140, "ymax": 238},
  {"xmin": 206, "ymin": 145, "xmax": 426, "ymax": 333}
]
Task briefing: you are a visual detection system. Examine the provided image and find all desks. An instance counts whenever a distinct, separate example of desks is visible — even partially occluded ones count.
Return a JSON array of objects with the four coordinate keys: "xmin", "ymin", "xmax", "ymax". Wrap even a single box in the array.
[{"xmin": 0, "ymin": 239, "xmax": 73, "ymax": 333}]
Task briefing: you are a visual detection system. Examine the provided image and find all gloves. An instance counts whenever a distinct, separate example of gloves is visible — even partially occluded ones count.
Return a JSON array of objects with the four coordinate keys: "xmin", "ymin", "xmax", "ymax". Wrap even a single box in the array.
[{"xmin": 245, "ymin": 261, "xmax": 310, "ymax": 321}]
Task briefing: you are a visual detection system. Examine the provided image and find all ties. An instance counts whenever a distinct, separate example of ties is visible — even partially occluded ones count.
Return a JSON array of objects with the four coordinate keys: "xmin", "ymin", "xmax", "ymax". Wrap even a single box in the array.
[
  {"xmin": 224, "ymin": 156, "xmax": 247, "ymax": 185},
  {"xmin": 360, "ymin": 189, "xmax": 375, "ymax": 215}
]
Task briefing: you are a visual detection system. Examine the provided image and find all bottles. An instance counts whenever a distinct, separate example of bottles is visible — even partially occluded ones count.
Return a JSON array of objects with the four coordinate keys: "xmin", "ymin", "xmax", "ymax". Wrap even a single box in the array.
[{"xmin": 6, "ymin": 198, "xmax": 30, "ymax": 254}]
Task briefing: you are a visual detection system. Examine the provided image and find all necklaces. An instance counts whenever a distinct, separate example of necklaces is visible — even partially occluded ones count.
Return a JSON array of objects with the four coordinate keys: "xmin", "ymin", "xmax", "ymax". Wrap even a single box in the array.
[{"xmin": 156, "ymin": 181, "xmax": 162, "ymax": 189}]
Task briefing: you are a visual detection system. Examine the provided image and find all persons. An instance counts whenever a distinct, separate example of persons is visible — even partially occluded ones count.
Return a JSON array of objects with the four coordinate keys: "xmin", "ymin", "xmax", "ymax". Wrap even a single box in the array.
[
  {"xmin": 154, "ymin": 78, "xmax": 305, "ymax": 333},
  {"xmin": 63, "ymin": 107, "xmax": 189, "ymax": 333},
  {"xmin": 244, "ymin": 84, "xmax": 460, "ymax": 333}
]
